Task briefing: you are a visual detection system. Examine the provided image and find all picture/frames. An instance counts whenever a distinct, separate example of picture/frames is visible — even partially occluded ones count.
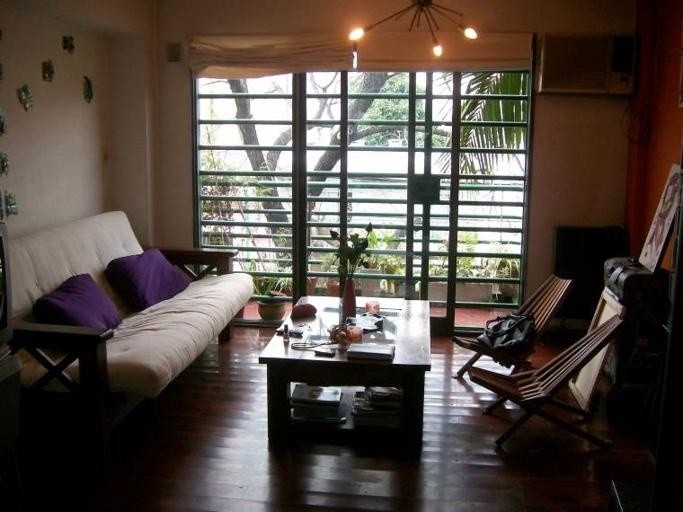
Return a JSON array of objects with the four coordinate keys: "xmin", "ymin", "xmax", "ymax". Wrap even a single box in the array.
[{"xmin": 568, "ymin": 288, "xmax": 626, "ymax": 412}]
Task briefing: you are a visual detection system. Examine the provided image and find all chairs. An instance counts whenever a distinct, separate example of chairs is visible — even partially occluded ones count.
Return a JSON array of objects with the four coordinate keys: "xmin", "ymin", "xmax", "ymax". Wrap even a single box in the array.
[
  {"xmin": 466, "ymin": 314, "xmax": 624, "ymax": 451},
  {"xmin": 452, "ymin": 274, "xmax": 574, "ymax": 398}
]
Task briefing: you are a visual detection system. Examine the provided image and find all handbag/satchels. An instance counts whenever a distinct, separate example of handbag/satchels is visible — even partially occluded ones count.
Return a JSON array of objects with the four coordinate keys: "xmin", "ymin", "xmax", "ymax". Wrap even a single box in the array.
[{"xmin": 478, "ymin": 312, "xmax": 537, "ymax": 369}]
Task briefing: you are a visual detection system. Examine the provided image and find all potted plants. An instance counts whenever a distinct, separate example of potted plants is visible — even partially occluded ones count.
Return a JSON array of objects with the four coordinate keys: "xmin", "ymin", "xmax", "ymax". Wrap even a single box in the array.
[
  {"xmin": 321, "ymin": 252, "xmax": 355, "ymax": 296},
  {"xmin": 330, "ymin": 222, "xmax": 372, "ymax": 323},
  {"xmin": 361, "ymin": 230, "xmax": 397, "ymax": 296},
  {"xmin": 251, "ymin": 276, "xmax": 293, "ymax": 321}
]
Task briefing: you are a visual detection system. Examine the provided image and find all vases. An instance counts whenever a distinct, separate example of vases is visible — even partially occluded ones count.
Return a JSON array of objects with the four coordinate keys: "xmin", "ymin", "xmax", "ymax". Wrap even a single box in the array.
[{"xmin": 306, "ymin": 277, "xmax": 318, "ymax": 296}]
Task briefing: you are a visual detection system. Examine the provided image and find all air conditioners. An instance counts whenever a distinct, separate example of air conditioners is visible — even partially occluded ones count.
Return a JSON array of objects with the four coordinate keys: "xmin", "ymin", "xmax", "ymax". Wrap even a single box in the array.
[{"xmin": 536, "ymin": 31, "xmax": 636, "ymax": 95}]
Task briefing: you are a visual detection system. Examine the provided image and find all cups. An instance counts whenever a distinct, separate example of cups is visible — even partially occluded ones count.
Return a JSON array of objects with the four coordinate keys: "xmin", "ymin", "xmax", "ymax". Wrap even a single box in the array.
[
  {"xmin": 366, "ymin": 300, "xmax": 379, "ymax": 313},
  {"xmin": 347, "ymin": 325, "xmax": 362, "ymax": 343}
]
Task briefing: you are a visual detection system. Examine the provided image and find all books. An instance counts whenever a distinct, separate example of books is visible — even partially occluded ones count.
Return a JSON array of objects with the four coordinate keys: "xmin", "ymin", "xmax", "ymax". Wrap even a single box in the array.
[
  {"xmin": 347, "ymin": 343, "xmax": 395, "ymax": 360},
  {"xmin": 290, "ymin": 383, "xmax": 404, "ymax": 423}
]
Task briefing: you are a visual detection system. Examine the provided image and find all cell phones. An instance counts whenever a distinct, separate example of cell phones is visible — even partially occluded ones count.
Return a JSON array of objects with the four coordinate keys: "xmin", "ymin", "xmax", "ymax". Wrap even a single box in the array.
[{"xmin": 314, "ymin": 347, "xmax": 335, "ymax": 356}]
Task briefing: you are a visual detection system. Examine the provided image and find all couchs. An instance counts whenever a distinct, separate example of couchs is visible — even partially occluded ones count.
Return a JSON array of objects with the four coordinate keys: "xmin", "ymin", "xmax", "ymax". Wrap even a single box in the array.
[{"xmin": 7, "ymin": 211, "xmax": 255, "ymax": 457}]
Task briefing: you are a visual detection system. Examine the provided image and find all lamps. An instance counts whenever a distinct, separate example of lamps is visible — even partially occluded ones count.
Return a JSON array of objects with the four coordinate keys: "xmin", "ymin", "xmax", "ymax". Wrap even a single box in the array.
[{"xmin": 348, "ymin": 0, "xmax": 478, "ymax": 57}]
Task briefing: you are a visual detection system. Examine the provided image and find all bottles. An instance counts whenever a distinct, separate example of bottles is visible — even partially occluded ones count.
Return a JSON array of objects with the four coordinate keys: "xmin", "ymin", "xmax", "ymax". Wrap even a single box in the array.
[{"xmin": 283, "ymin": 324, "xmax": 290, "ymax": 343}]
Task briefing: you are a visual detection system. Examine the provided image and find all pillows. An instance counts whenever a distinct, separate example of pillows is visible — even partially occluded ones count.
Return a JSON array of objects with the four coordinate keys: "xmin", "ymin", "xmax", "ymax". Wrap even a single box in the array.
[
  {"xmin": 32, "ymin": 274, "xmax": 123, "ymax": 357},
  {"xmin": 104, "ymin": 248, "xmax": 193, "ymax": 313}
]
